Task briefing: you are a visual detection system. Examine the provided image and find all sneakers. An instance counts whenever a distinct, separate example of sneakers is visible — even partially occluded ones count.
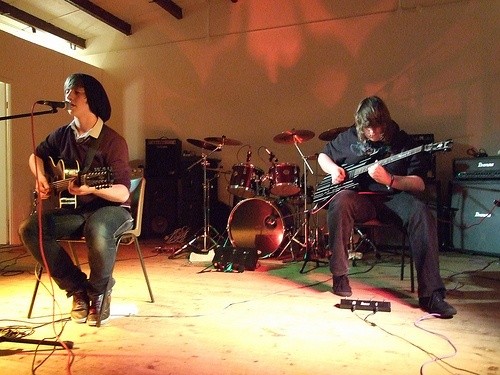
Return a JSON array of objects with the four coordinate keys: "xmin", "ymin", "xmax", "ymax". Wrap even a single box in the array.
[
  {"xmin": 333, "ymin": 274, "xmax": 352, "ymax": 297},
  {"xmin": 419, "ymin": 291, "xmax": 457, "ymax": 318},
  {"xmin": 70, "ymin": 272, "xmax": 90, "ymax": 323},
  {"xmin": 86, "ymin": 288, "xmax": 112, "ymax": 325}
]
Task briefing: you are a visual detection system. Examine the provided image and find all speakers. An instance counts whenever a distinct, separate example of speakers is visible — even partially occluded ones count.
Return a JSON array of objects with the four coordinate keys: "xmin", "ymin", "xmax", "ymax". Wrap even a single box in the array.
[
  {"xmin": 143, "ymin": 137, "xmax": 218, "ymax": 241},
  {"xmin": 369, "ymin": 178, "xmax": 442, "ymax": 250},
  {"xmin": 447, "ymin": 179, "xmax": 500, "ymax": 258}
]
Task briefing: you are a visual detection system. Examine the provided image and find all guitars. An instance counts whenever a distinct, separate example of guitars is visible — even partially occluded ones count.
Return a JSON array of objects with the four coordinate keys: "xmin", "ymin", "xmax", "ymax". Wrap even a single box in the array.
[
  {"xmin": 313, "ymin": 138, "xmax": 453, "ymax": 203},
  {"xmin": 31, "ymin": 156, "xmax": 112, "ymax": 209}
]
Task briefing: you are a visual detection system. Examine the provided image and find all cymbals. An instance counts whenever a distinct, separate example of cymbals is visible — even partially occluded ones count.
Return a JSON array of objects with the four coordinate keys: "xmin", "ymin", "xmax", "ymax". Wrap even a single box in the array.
[
  {"xmin": 318, "ymin": 127, "xmax": 349, "ymax": 141},
  {"xmin": 187, "ymin": 139, "xmax": 221, "ymax": 151},
  {"xmin": 273, "ymin": 130, "xmax": 315, "ymax": 144},
  {"xmin": 204, "ymin": 137, "xmax": 243, "ymax": 146}
]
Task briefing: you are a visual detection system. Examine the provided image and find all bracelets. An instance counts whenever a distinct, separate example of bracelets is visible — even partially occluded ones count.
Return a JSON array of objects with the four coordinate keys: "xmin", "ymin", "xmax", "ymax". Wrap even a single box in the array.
[{"xmin": 390, "ymin": 175, "xmax": 394, "ymax": 187}]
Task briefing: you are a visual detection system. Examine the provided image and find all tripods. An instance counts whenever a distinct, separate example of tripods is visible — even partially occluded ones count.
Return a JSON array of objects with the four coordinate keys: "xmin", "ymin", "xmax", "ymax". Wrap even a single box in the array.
[
  {"xmin": 169, "ymin": 142, "xmax": 227, "ymax": 260},
  {"xmin": 274, "ymin": 138, "xmax": 330, "ymax": 273}
]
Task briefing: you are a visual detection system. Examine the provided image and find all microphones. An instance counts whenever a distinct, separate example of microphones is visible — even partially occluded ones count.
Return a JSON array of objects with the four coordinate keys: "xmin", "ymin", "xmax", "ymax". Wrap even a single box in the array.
[
  {"xmin": 267, "ymin": 218, "xmax": 275, "ymax": 225},
  {"xmin": 247, "ymin": 147, "xmax": 251, "ymax": 161},
  {"xmin": 265, "ymin": 147, "xmax": 278, "ymax": 162},
  {"xmin": 36, "ymin": 100, "xmax": 71, "ymax": 110}
]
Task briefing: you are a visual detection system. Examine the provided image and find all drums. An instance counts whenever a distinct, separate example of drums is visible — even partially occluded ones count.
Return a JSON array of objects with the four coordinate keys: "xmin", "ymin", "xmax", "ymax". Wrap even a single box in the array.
[
  {"xmin": 269, "ymin": 161, "xmax": 301, "ymax": 197},
  {"xmin": 225, "ymin": 196, "xmax": 294, "ymax": 259},
  {"xmin": 280, "ymin": 183, "xmax": 314, "ymax": 206},
  {"xmin": 229, "ymin": 162, "xmax": 264, "ymax": 198}
]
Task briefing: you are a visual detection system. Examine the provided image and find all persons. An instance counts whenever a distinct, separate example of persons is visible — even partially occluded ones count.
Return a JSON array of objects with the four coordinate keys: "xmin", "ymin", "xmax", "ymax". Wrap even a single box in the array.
[
  {"xmin": 19, "ymin": 74, "xmax": 132, "ymax": 326},
  {"xmin": 317, "ymin": 96, "xmax": 458, "ymax": 316}
]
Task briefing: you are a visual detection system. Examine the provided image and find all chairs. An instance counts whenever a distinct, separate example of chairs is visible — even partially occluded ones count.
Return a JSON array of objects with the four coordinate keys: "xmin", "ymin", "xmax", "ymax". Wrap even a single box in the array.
[
  {"xmin": 28, "ymin": 178, "xmax": 155, "ymax": 327},
  {"xmin": 357, "ymin": 217, "xmax": 415, "ymax": 292}
]
